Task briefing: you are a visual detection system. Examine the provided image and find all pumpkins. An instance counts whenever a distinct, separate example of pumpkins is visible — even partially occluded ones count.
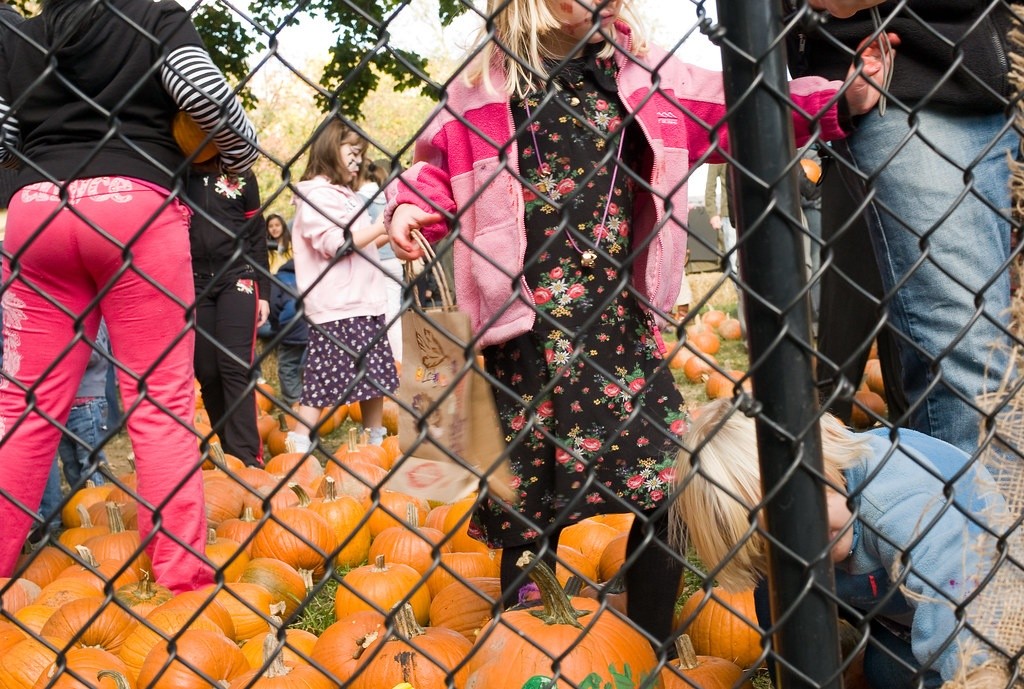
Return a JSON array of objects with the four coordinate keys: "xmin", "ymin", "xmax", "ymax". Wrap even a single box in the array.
[
  {"xmin": 174, "ymin": 108, "xmax": 218, "ymax": 163},
  {"xmin": 0, "ymin": 309, "xmax": 883, "ymax": 689},
  {"xmin": 798, "ymin": 158, "xmax": 821, "ymax": 183}
]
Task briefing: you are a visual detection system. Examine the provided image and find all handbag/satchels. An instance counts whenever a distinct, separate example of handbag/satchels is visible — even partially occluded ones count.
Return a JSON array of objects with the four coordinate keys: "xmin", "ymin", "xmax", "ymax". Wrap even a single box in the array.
[{"xmin": 398, "ymin": 227, "xmax": 511, "ymax": 469}]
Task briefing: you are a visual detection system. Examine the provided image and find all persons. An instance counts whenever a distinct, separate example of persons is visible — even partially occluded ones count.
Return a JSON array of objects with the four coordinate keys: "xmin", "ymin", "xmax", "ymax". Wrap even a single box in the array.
[
  {"xmin": 668, "ymin": 392, "xmax": 1016, "ymax": 689},
  {"xmin": 0, "ymin": 0, "xmax": 311, "ymax": 590},
  {"xmin": 288, "ymin": 113, "xmax": 398, "ymax": 464},
  {"xmin": 780, "ymin": 0, "xmax": 1024, "ymax": 464},
  {"xmin": 380, "ymin": 0, "xmax": 903, "ymax": 666}
]
